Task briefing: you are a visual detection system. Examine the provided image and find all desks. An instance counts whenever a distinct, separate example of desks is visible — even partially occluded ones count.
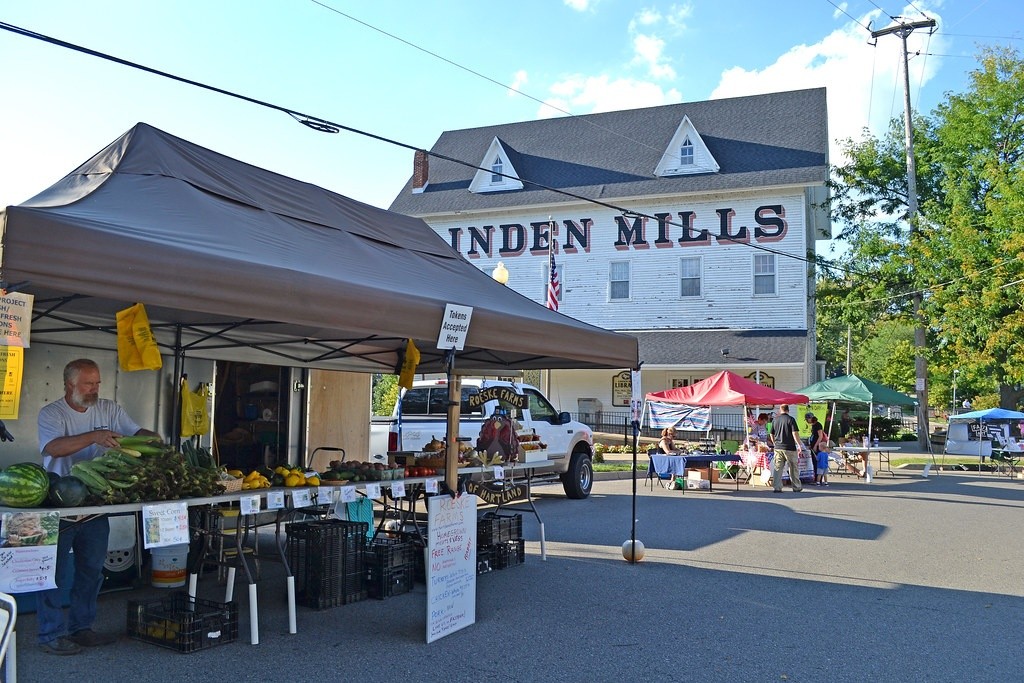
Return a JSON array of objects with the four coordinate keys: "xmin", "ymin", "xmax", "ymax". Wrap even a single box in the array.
[
  {"xmin": 436, "ymin": 460, "xmax": 555, "ymax": 562},
  {"xmin": 0, "ymin": 475, "xmax": 445, "ymax": 645},
  {"xmin": 833, "ymin": 447, "xmax": 902, "ymax": 480},
  {"xmin": 990, "ymin": 448, "xmax": 1024, "ymax": 479},
  {"xmin": 730, "ymin": 450, "xmax": 815, "ymax": 486},
  {"xmin": 651, "ymin": 453, "xmax": 743, "ymax": 495}
]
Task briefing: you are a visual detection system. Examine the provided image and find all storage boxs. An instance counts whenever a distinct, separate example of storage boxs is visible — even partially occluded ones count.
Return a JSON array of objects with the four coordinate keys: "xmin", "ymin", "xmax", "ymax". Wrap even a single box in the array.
[
  {"xmin": 676, "ymin": 467, "xmax": 724, "ymax": 490},
  {"xmin": 477, "ymin": 514, "xmax": 525, "ymax": 573},
  {"xmin": 285, "ymin": 518, "xmax": 429, "ymax": 610},
  {"xmin": 187, "ymin": 504, "xmax": 222, "ymax": 573},
  {"xmin": 127, "ymin": 588, "xmax": 239, "ymax": 653},
  {"xmin": 387, "ymin": 451, "xmax": 415, "ymax": 467}
]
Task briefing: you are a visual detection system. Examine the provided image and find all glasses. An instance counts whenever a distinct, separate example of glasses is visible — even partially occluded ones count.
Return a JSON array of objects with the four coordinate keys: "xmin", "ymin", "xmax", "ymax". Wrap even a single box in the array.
[{"xmin": 673, "ymin": 436, "xmax": 676, "ymax": 437}]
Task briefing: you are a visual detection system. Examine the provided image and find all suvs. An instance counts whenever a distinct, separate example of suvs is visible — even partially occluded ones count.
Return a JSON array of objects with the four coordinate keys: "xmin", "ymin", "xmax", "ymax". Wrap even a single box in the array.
[{"xmin": 389, "ymin": 379, "xmax": 597, "ymax": 499}]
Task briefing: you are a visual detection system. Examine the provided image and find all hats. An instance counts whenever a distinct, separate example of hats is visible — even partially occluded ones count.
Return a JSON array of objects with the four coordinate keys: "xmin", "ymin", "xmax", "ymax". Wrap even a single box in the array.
[{"xmin": 804, "ymin": 413, "xmax": 813, "ymax": 421}]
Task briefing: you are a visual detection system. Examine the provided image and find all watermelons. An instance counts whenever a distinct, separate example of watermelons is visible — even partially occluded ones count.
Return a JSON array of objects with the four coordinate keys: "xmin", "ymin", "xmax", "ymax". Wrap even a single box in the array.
[{"xmin": 0, "ymin": 462, "xmax": 88, "ymax": 508}]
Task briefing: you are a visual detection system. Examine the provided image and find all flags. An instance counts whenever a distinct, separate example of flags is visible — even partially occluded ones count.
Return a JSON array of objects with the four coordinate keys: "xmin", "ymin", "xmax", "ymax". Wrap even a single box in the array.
[{"xmin": 547, "ymin": 240, "xmax": 560, "ymax": 312}]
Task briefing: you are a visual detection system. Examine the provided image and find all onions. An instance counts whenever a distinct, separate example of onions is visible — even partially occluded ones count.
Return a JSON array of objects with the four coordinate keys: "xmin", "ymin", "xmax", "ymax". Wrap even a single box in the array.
[{"xmin": 422, "ymin": 435, "xmax": 466, "ymax": 453}]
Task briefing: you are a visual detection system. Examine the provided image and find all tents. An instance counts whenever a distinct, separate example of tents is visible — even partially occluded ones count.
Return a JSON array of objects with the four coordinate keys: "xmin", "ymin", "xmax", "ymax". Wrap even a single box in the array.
[
  {"xmin": 0, "ymin": 122, "xmax": 642, "ymax": 568},
  {"xmin": 788, "ymin": 373, "xmax": 941, "ymax": 479},
  {"xmin": 941, "ymin": 401, "xmax": 1024, "ymax": 475},
  {"xmin": 628, "ymin": 371, "xmax": 809, "ymax": 488}
]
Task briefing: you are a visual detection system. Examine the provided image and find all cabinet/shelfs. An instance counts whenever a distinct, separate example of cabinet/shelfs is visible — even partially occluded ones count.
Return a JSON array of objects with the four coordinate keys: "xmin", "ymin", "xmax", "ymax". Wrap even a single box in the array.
[
  {"xmin": 234, "ymin": 364, "xmax": 286, "ymax": 433},
  {"xmin": 514, "ymin": 428, "xmax": 547, "ymax": 463}
]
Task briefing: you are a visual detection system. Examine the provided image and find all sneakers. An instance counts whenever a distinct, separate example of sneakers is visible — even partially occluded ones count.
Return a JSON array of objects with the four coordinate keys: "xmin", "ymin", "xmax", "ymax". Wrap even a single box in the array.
[
  {"xmin": 69, "ymin": 630, "xmax": 115, "ymax": 646},
  {"xmin": 37, "ymin": 636, "xmax": 81, "ymax": 654}
]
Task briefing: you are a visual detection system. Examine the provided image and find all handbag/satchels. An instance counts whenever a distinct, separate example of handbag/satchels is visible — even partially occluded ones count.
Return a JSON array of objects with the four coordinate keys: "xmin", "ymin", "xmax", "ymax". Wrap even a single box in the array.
[{"xmin": 180, "ymin": 380, "xmax": 209, "ymax": 438}]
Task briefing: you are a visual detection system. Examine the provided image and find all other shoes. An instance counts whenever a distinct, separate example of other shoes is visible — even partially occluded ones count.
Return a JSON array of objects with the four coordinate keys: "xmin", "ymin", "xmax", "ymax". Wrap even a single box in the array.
[
  {"xmin": 793, "ymin": 488, "xmax": 802, "ymax": 492},
  {"xmin": 817, "ymin": 481, "xmax": 829, "ymax": 487},
  {"xmin": 773, "ymin": 490, "xmax": 783, "ymax": 493}
]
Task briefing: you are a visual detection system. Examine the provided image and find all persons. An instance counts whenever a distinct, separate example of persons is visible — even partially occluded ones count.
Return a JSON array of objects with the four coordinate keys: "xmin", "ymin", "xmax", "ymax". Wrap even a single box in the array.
[
  {"xmin": 962, "ymin": 398, "xmax": 972, "ymax": 409},
  {"xmin": 1016, "ymin": 419, "xmax": 1024, "ymax": 440},
  {"xmin": 658, "ymin": 427, "xmax": 681, "ymax": 480},
  {"xmin": 35, "ymin": 359, "xmax": 163, "ymax": 656},
  {"xmin": 769, "ymin": 403, "xmax": 803, "ymax": 492},
  {"xmin": 841, "ymin": 407, "xmax": 851, "ymax": 435},
  {"xmin": 934, "ymin": 408, "xmax": 939, "ymax": 421},
  {"xmin": 845, "ymin": 435, "xmax": 867, "ymax": 477},
  {"xmin": 944, "ymin": 414, "xmax": 949, "ymax": 423},
  {"xmin": 815, "ymin": 442, "xmax": 829, "ymax": 487},
  {"xmin": 804, "ymin": 412, "xmax": 823, "ymax": 486},
  {"xmin": 750, "ymin": 413, "xmax": 774, "ymax": 446}
]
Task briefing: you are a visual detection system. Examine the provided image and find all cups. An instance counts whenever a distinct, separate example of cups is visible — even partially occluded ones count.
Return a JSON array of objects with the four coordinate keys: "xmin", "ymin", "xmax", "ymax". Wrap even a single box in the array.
[{"xmin": 874, "ymin": 439, "xmax": 879, "ymax": 447}]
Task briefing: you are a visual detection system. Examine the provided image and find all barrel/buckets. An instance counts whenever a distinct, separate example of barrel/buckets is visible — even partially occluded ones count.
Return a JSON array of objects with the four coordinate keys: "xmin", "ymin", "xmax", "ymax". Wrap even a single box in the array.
[{"xmin": 154, "ymin": 544, "xmax": 188, "ymax": 587}]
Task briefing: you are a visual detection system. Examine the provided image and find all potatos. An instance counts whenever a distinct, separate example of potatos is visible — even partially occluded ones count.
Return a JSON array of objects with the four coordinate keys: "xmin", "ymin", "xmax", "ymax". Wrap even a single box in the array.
[{"xmin": 329, "ymin": 459, "xmax": 398, "ymax": 471}]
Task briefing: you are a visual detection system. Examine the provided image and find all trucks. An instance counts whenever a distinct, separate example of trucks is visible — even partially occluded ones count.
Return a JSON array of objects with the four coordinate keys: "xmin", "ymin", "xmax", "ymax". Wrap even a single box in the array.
[{"xmin": 1, "ymin": 343, "xmax": 374, "ymax": 584}]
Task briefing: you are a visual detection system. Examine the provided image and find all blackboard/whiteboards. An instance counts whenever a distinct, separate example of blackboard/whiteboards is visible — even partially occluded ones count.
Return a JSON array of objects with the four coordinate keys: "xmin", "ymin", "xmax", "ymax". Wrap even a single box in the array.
[{"xmin": 427, "ymin": 493, "xmax": 478, "ymax": 643}]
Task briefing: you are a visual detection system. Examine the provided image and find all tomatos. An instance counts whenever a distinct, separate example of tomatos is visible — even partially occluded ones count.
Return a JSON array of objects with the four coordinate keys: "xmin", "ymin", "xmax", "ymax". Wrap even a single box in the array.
[{"xmin": 403, "ymin": 466, "xmax": 437, "ymax": 477}]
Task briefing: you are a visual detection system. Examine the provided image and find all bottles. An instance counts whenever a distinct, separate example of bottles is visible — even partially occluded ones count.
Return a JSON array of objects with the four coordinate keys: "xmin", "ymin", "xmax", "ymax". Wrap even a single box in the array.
[{"xmin": 716, "ymin": 436, "xmax": 721, "ymax": 455}]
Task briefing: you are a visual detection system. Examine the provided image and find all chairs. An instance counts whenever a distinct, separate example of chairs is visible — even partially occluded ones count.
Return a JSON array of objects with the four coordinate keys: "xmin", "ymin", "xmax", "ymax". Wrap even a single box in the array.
[
  {"xmin": 286, "ymin": 447, "xmax": 346, "ymax": 521},
  {"xmin": 0, "ymin": 592, "xmax": 17, "ymax": 683},
  {"xmin": 722, "ymin": 440, "xmax": 748, "ymax": 481},
  {"xmin": 645, "ymin": 448, "xmax": 669, "ymax": 488},
  {"xmin": 828, "ymin": 437, "xmax": 863, "ymax": 476}
]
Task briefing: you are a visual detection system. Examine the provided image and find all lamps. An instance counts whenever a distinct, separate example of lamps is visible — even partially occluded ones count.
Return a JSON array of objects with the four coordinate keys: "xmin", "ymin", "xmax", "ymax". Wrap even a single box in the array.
[{"xmin": 721, "ymin": 349, "xmax": 729, "ymax": 357}]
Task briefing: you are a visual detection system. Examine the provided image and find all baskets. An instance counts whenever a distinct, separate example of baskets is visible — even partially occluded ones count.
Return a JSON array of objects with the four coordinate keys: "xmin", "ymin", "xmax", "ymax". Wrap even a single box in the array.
[
  {"xmin": 214, "ymin": 478, "xmax": 243, "ymax": 493},
  {"xmin": 321, "ymin": 480, "xmax": 349, "ymax": 485}
]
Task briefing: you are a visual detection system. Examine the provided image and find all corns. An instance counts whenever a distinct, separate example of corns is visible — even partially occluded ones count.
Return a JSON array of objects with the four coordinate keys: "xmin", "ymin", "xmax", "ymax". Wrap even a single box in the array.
[{"xmin": 67, "ymin": 434, "xmax": 226, "ymax": 505}]
